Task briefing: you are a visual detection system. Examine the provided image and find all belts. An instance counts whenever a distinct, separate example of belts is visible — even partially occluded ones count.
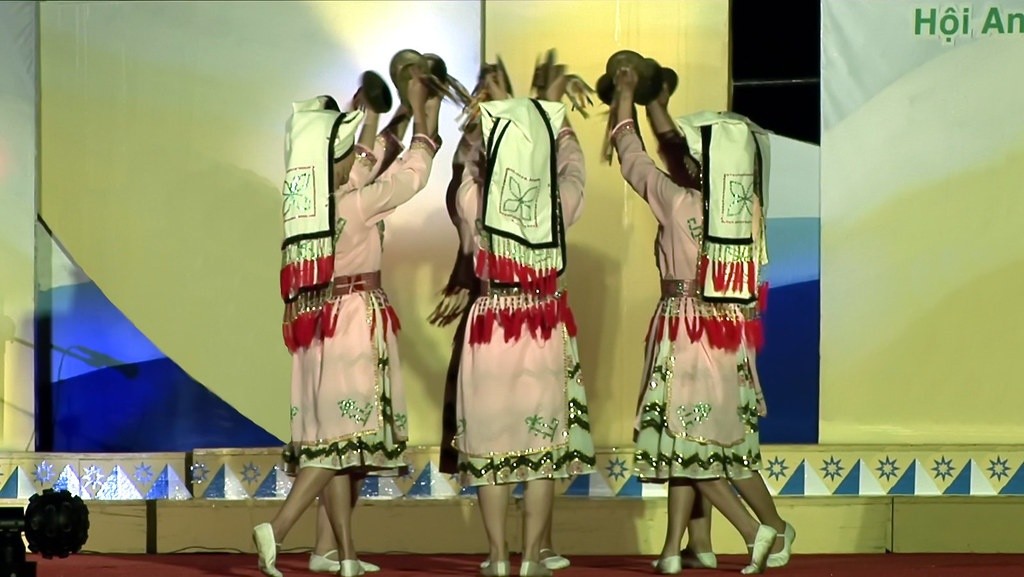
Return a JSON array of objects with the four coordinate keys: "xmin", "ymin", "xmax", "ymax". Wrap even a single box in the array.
[
  {"xmin": 479, "ymin": 282, "xmax": 488, "ymax": 295},
  {"xmin": 333, "ymin": 271, "xmax": 381, "ymax": 295},
  {"xmin": 661, "ymin": 279, "xmax": 698, "ymax": 298}
]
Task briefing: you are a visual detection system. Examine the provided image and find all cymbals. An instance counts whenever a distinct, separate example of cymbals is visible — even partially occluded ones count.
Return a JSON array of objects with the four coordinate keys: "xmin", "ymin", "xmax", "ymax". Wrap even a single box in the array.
[
  {"xmin": 360, "ymin": 49, "xmax": 448, "ymax": 114},
  {"xmin": 595, "ymin": 50, "xmax": 679, "ymax": 104},
  {"xmin": 479, "ymin": 48, "xmax": 562, "ymax": 98}
]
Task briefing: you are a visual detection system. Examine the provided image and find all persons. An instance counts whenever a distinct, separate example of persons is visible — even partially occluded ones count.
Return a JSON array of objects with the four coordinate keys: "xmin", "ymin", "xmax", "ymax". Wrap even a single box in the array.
[
  {"xmin": 253, "ymin": 57, "xmax": 597, "ymax": 577},
  {"xmin": 608, "ymin": 65, "xmax": 797, "ymax": 574}
]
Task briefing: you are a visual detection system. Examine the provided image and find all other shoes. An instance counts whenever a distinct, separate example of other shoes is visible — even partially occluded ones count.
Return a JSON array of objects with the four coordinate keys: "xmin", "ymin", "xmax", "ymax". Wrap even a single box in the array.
[
  {"xmin": 252, "ymin": 523, "xmax": 283, "ymax": 577},
  {"xmin": 741, "ymin": 525, "xmax": 777, "ymax": 575},
  {"xmin": 309, "ymin": 550, "xmax": 382, "ymax": 572},
  {"xmin": 538, "ymin": 548, "xmax": 570, "ymax": 570},
  {"xmin": 682, "ymin": 552, "xmax": 717, "ymax": 568},
  {"xmin": 652, "ymin": 556, "xmax": 680, "ymax": 574},
  {"xmin": 519, "ymin": 561, "xmax": 553, "ymax": 577},
  {"xmin": 340, "ymin": 559, "xmax": 365, "ymax": 577},
  {"xmin": 766, "ymin": 522, "xmax": 796, "ymax": 568},
  {"xmin": 480, "ymin": 560, "xmax": 509, "ymax": 577}
]
